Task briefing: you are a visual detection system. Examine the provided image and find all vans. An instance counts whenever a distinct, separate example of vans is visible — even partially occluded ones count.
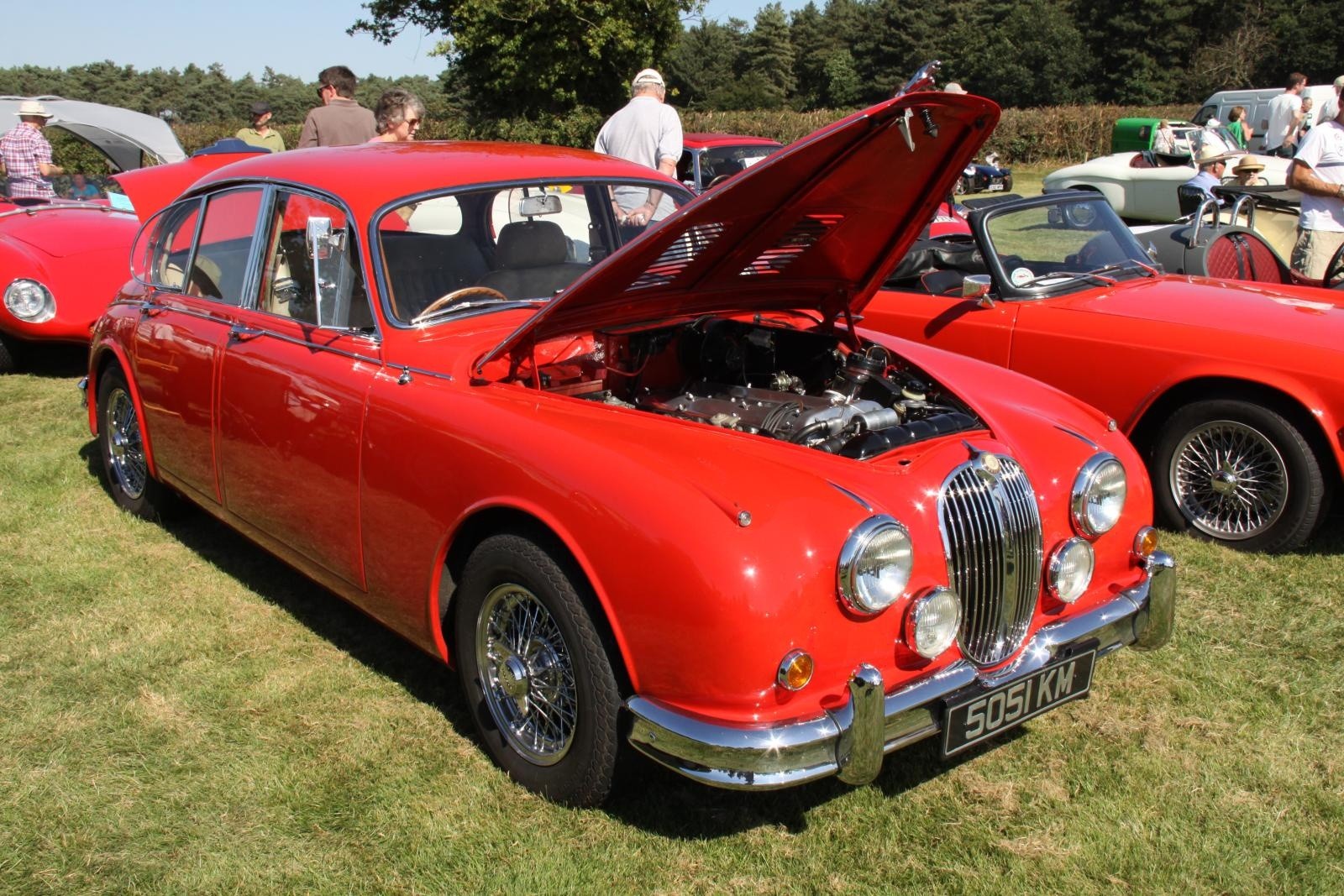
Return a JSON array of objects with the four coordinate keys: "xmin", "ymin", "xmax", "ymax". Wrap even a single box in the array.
[{"xmin": 1189, "ymin": 84, "xmax": 1341, "ymax": 157}]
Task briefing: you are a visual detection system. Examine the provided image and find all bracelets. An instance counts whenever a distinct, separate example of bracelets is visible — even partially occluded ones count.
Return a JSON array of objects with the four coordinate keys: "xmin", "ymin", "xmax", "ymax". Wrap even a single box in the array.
[{"xmin": 1337, "ymin": 184, "xmax": 1344, "ymax": 198}]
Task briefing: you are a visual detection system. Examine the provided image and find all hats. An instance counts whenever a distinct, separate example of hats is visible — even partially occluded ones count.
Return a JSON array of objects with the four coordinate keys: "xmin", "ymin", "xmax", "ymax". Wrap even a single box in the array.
[
  {"xmin": 1232, "ymin": 157, "xmax": 1266, "ymax": 176},
  {"xmin": 1193, "ymin": 146, "xmax": 1237, "ymax": 164},
  {"xmin": 632, "ymin": 68, "xmax": 666, "ymax": 88},
  {"xmin": 249, "ymin": 102, "xmax": 271, "ymax": 114},
  {"xmin": 945, "ymin": 82, "xmax": 968, "ymax": 95},
  {"xmin": 13, "ymin": 100, "xmax": 53, "ymax": 118}
]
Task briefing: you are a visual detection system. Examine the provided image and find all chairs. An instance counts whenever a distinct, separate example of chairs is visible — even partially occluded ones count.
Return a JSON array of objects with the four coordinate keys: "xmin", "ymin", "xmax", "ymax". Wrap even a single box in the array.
[
  {"xmin": 493, "ymin": 219, "xmax": 587, "ymax": 296},
  {"xmin": 714, "ymin": 159, "xmax": 743, "ymax": 176},
  {"xmin": 269, "ymin": 231, "xmax": 374, "ymax": 331},
  {"xmin": 1134, "ymin": 160, "xmax": 1152, "ymax": 167},
  {"xmin": 918, "ymin": 267, "xmax": 972, "ymax": 296}
]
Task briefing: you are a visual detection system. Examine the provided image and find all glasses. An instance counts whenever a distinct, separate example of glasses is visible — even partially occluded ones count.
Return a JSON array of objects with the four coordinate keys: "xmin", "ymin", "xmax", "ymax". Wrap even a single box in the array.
[
  {"xmin": 316, "ymin": 83, "xmax": 331, "ymax": 98},
  {"xmin": 1240, "ymin": 169, "xmax": 1259, "ymax": 174},
  {"xmin": 404, "ymin": 117, "xmax": 422, "ymax": 126}
]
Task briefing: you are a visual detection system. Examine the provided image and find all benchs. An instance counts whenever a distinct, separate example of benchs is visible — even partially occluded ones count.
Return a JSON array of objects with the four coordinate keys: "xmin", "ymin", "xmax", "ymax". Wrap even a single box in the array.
[{"xmin": 171, "ymin": 232, "xmax": 478, "ymax": 323}]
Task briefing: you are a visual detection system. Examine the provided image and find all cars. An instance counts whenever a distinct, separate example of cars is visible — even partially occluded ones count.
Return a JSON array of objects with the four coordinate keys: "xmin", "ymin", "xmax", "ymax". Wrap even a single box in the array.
[
  {"xmin": 401, "ymin": 133, "xmax": 784, "ymax": 266},
  {"xmin": 645, "ymin": 191, "xmax": 1344, "ymax": 560},
  {"xmin": 1065, "ymin": 195, "xmax": 1344, "ymax": 294},
  {"xmin": 78, "ymin": 58, "xmax": 1180, "ymax": 815},
  {"xmin": 0, "ymin": 192, "xmax": 154, "ymax": 348},
  {"xmin": 954, "ymin": 164, "xmax": 1014, "ymax": 196},
  {"xmin": 1043, "ymin": 125, "xmax": 1305, "ymax": 230}
]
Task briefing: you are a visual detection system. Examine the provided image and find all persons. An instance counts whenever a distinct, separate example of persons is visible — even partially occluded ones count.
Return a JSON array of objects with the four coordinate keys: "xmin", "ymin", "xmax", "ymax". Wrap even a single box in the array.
[
  {"xmin": 68, "ymin": 172, "xmax": 101, "ymax": 201},
  {"xmin": 237, "ymin": 102, "xmax": 286, "ymax": 153},
  {"xmin": 1290, "ymin": 88, "xmax": 1344, "ymax": 280},
  {"xmin": 1224, "ymin": 155, "xmax": 1265, "ymax": 187},
  {"xmin": 1204, "ymin": 114, "xmax": 1221, "ymax": 127},
  {"xmin": 1188, "ymin": 144, "xmax": 1239, "ymax": 205},
  {"xmin": 368, "ymin": 88, "xmax": 426, "ymax": 143},
  {"xmin": 1226, "ymin": 106, "xmax": 1254, "ymax": 154},
  {"xmin": 1152, "ymin": 118, "xmax": 1175, "ymax": 155},
  {"xmin": 297, "ymin": 66, "xmax": 380, "ymax": 149},
  {"xmin": 593, "ymin": 68, "xmax": 683, "ymax": 247},
  {"xmin": 0, "ymin": 101, "xmax": 63, "ymax": 207},
  {"xmin": 1262, "ymin": 73, "xmax": 1344, "ymax": 160}
]
